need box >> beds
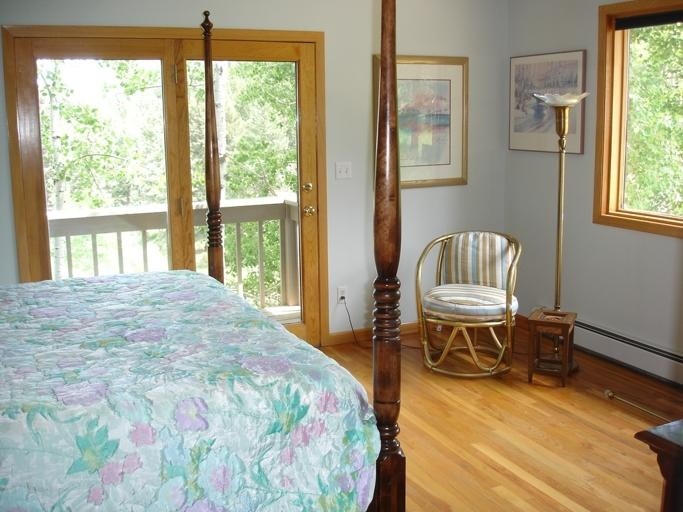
[0,2,407,512]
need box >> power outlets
[335,284,347,305]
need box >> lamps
[527,87,589,381]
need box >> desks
[633,418,682,512]
[526,307,577,387]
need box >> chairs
[414,229,523,381]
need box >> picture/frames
[370,53,471,192]
[507,49,586,155]
